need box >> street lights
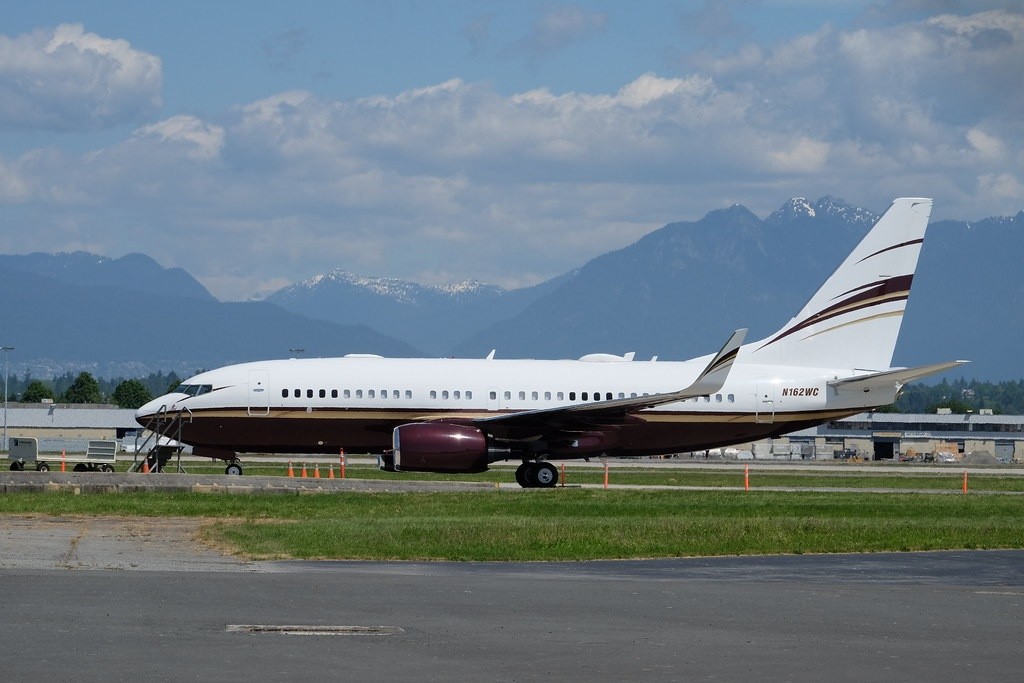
[0,345,16,452]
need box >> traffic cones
[143,455,149,473]
[327,462,335,478]
[288,459,295,477]
[313,461,320,477]
[301,461,309,478]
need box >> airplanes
[132,196,972,490]
